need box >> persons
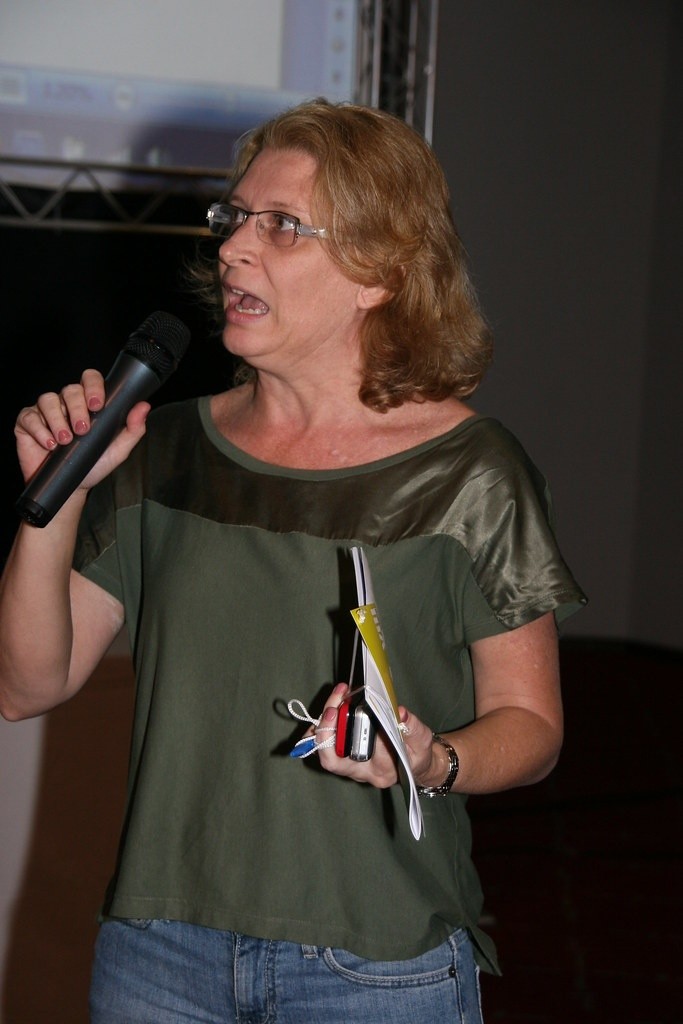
[0,99,589,1024]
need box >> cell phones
[337,696,357,757]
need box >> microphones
[16,311,190,528]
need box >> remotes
[352,698,378,762]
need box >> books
[353,547,426,842]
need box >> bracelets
[418,734,460,798]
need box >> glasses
[206,203,331,248]
